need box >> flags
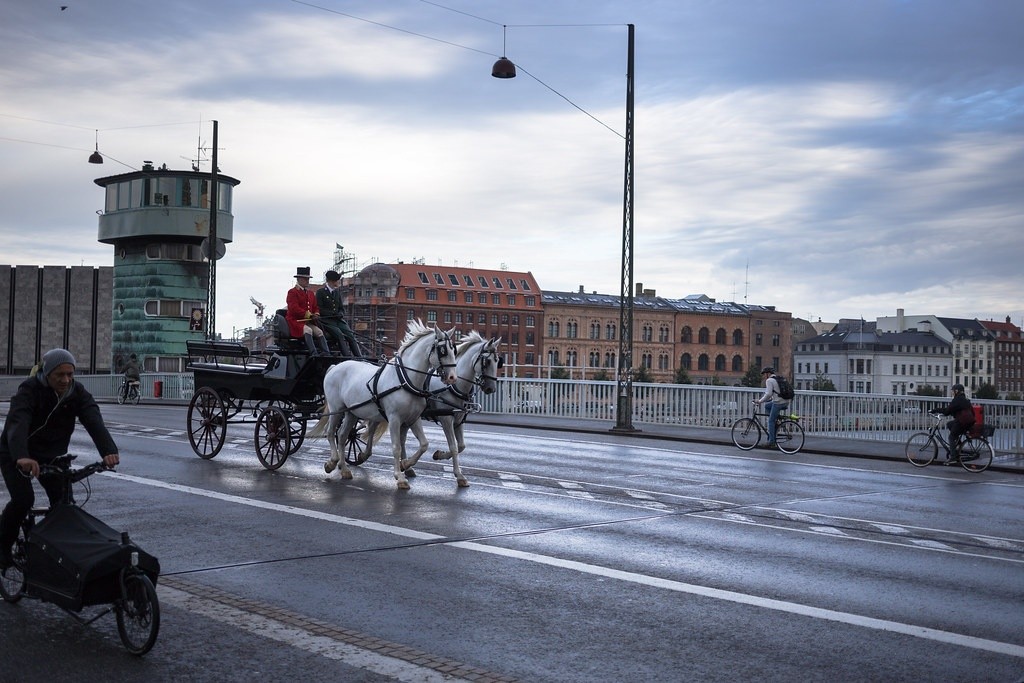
[337,243,343,249]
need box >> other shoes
[0,546,14,569]
[763,444,776,449]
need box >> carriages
[184,318,501,495]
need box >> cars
[905,406,921,413]
[712,401,737,412]
[515,401,541,413]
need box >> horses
[359,329,502,488]
[307,317,458,488]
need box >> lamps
[88,152,103,164]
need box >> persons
[120,353,141,398]
[0,347,120,571]
[754,367,789,447]
[316,271,362,357]
[286,267,330,355]
[927,384,976,464]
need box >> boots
[336,333,362,357]
[303,333,331,357]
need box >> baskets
[973,424,995,437]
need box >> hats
[760,367,773,374]
[326,271,341,282]
[294,267,313,278]
[43,349,75,377]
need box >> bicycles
[905,410,996,474]
[731,402,805,456]
[115,372,141,406]
[0,460,160,658]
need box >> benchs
[185,309,336,374]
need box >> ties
[332,291,334,298]
[304,288,307,292]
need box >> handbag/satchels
[272,314,289,336]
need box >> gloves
[338,312,344,318]
[310,314,318,326]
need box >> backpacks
[764,376,794,399]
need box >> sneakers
[944,459,961,466]
[123,394,127,398]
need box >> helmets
[950,384,964,392]
[129,353,137,359]
